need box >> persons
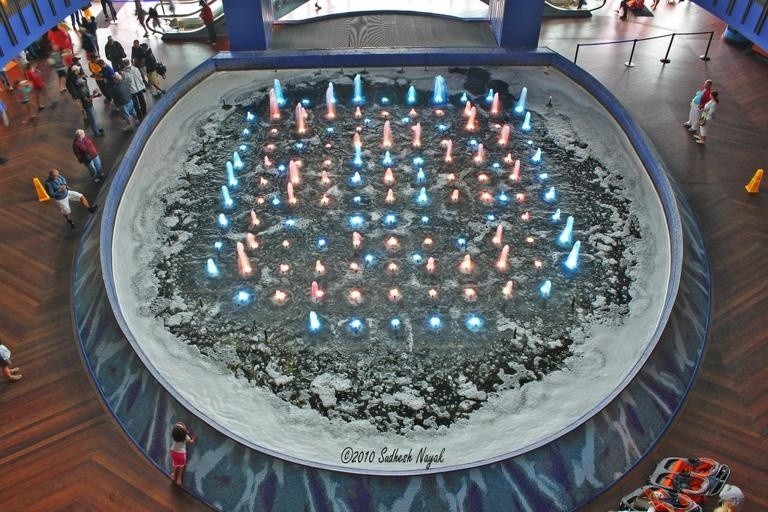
[0,341,22,380]
[72,129,105,184]
[198,0,216,46]
[168,421,196,486]
[693,90,719,144]
[619,0,628,22]
[44,167,98,229]
[0,1,168,139]
[713,483,745,512]
[682,79,713,131]
[577,0,587,10]
[650,0,660,11]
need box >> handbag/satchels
[155,62,167,74]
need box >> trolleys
[619,454,731,511]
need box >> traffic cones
[32,177,50,203]
[744,164,763,194]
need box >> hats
[71,27,148,85]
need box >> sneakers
[135,120,140,126]
[152,90,161,96]
[90,204,98,214]
[161,89,166,94]
[100,173,104,176]
[22,114,36,124]
[94,178,100,182]
[60,87,67,92]
[38,105,45,110]
[68,219,74,229]
[123,125,133,132]
[51,100,59,105]
[95,134,104,137]
[685,123,708,150]
[99,127,104,133]
